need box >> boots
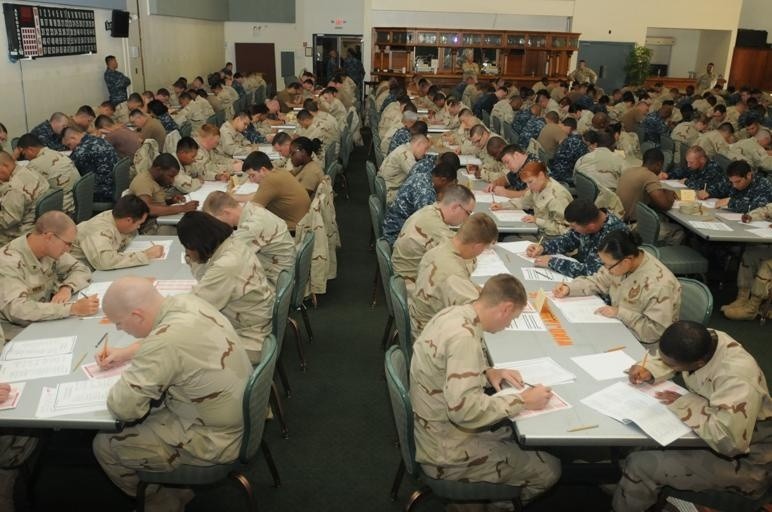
[445,499,484,512]
[142,483,195,511]
[719,286,761,322]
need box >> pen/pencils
[746,205,751,223]
[638,348,650,385]
[530,236,543,258]
[102,336,107,361]
[703,182,707,200]
[95,332,108,349]
[603,345,627,353]
[73,352,88,372]
[188,193,193,202]
[80,292,88,299]
[521,380,535,388]
[535,271,553,280]
[492,191,495,204]
[568,423,599,432]
[150,240,155,246]
[505,254,511,263]
[558,279,564,297]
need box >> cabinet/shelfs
[365,26,583,81]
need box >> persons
[0,52,367,512]
[375,59,772,512]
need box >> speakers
[111,8,129,38]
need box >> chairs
[251,268,294,409]
[0,67,772,332]
[381,343,523,511]
[128,336,281,509]
[289,232,317,365]
[663,483,745,511]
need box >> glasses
[44,230,73,249]
[602,253,631,272]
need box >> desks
[1,93,317,433]
[390,98,717,451]
